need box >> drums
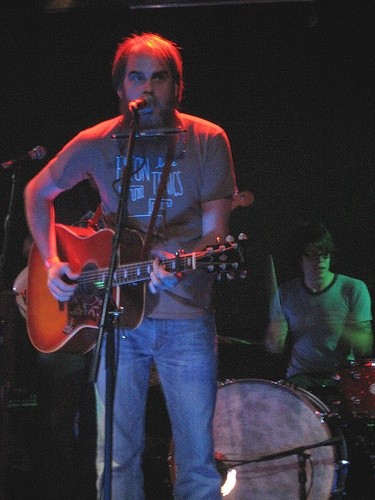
[167,376,351,500]
[330,358,375,424]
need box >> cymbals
[216,335,266,347]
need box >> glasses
[302,251,332,262]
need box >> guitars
[25,222,253,356]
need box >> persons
[25,35,240,500]
[265,223,374,380]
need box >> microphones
[0,146,47,170]
[129,94,155,112]
